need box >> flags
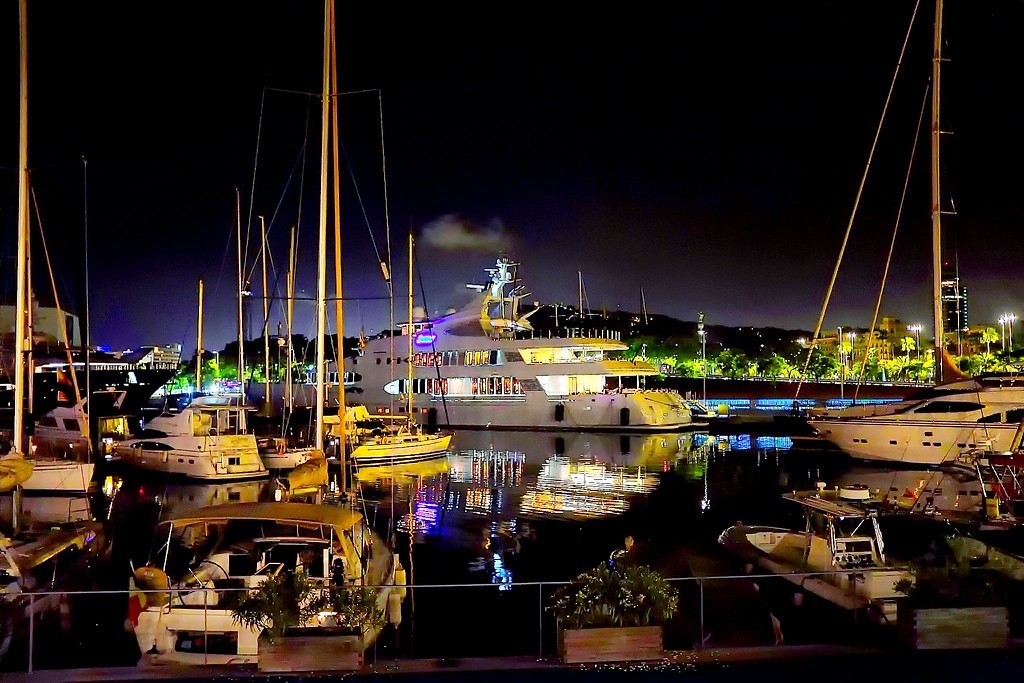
[57,369,73,402]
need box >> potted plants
[228,565,386,673]
[543,561,679,664]
[894,557,1012,650]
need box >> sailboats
[771,1,1023,469]
[0,0,454,683]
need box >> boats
[245,252,711,435]
[716,480,932,630]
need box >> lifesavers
[384,438,387,443]
[276,443,286,455]
